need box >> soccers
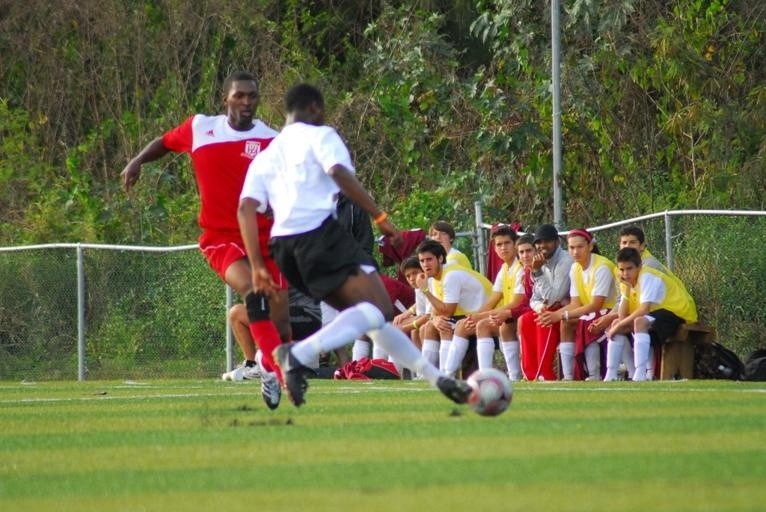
[464,368,514,417]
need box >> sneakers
[221,367,243,382]
[271,341,312,408]
[254,348,287,411]
[435,375,475,403]
[243,360,259,380]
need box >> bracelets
[408,306,416,315]
[560,307,570,321]
[373,212,387,226]
[427,317,433,320]
[421,288,429,294]
[413,321,418,330]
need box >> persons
[221,281,322,381]
[119,72,294,393]
[417,221,697,383]
[332,254,416,380]
[237,83,474,407]
[318,193,378,378]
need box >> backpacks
[742,347,765,382]
[692,339,744,380]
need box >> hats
[531,223,558,245]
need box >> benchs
[459,322,715,382]
